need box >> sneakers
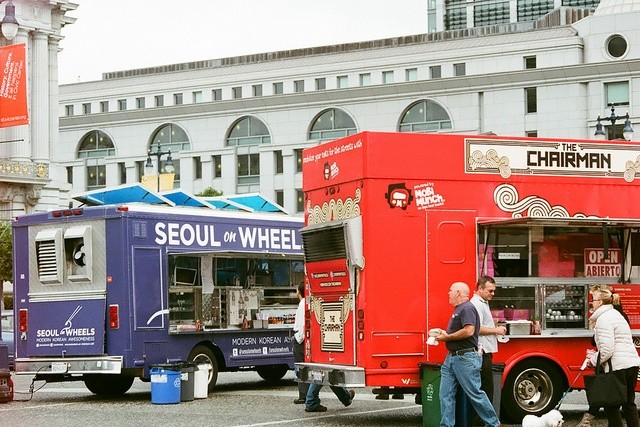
[376,396,388,399]
[294,399,306,404]
[392,395,404,399]
[305,406,327,412]
[346,390,355,406]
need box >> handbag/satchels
[583,350,627,408]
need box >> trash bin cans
[491,363,505,421]
[148,363,182,404]
[180,363,199,402]
[194,363,213,398]
[418,362,442,427]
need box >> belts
[450,347,476,356]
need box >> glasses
[487,290,497,293]
[592,297,596,302]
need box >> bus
[294,130,640,427]
[11,202,306,396]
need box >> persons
[304,383,355,411]
[470,276,507,427]
[433,282,503,427]
[586,289,639,426]
[574,283,631,427]
[293,281,308,404]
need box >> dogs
[522,409,565,427]
